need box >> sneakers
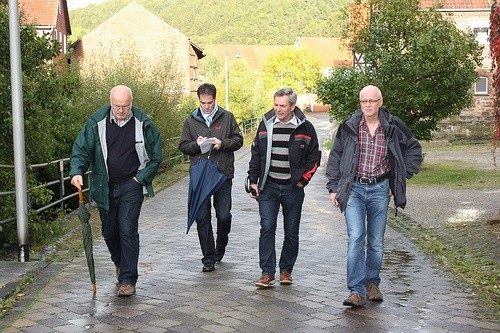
[117,283,135,296]
[343,293,366,306]
[115,268,120,279]
[255,276,276,287]
[365,283,384,301]
[279,272,292,283]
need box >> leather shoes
[215,244,226,262]
[203,262,214,272]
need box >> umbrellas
[76,181,97,294]
[244,177,258,204]
[186,143,227,236]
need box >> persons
[180,83,244,272]
[247,89,321,286]
[70,85,163,295]
[325,86,422,304]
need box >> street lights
[224,42,242,111]
[278,63,291,89]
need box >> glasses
[359,98,382,106]
[111,103,130,110]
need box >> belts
[354,171,392,185]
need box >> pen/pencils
[197,134,200,136]
